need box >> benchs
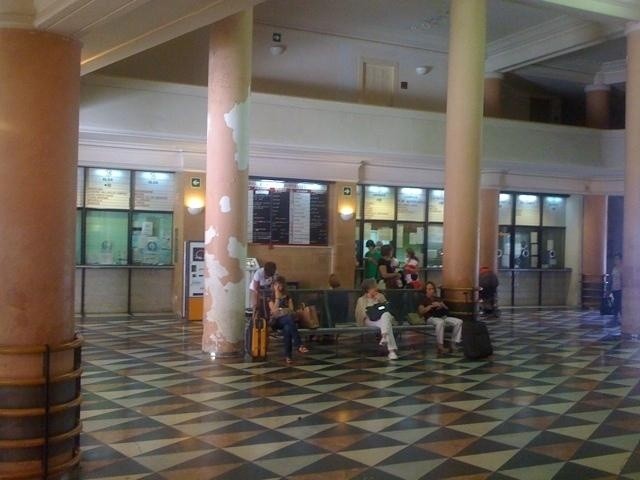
[251,286,453,357]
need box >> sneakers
[286,357,292,364]
[388,352,399,360]
[299,345,309,352]
[437,343,459,354]
[379,338,388,347]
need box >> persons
[418,281,463,354]
[250,260,281,319]
[267,276,310,363]
[356,239,499,321]
[606,253,622,317]
[354,277,399,360]
[310,274,349,344]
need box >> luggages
[246,311,267,362]
[462,293,493,359]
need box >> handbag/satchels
[600,292,618,315]
[296,302,319,328]
[407,310,425,325]
[432,307,448,318]
[404,258,420,274]
